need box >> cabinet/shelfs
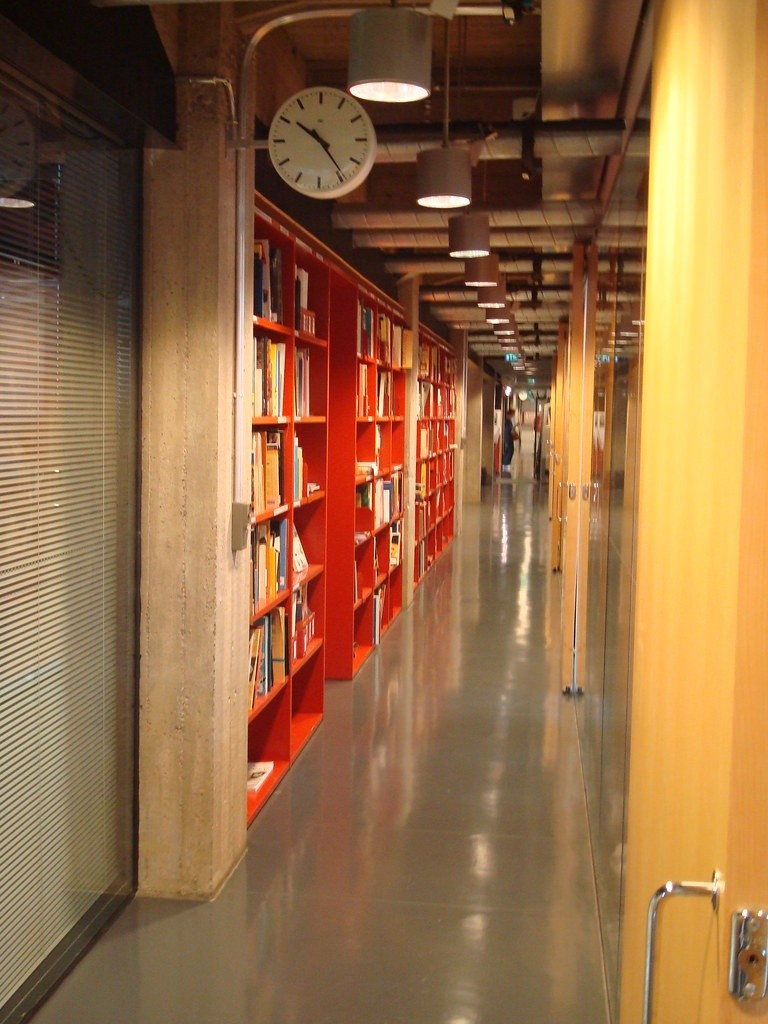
[409,318,459,589]
[244,191,332,827]
[322,260,405,679]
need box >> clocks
[267,87,378,200]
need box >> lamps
[476,274,505,308]
[485,300,509,324]
[497,325,525,370]
[347,7,432,104]
[493,313,515,336]
[464,253,498,286]
[415,16,472,209]
[448,206,489,258]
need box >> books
[250,228,455,708]
[247,761,274,791]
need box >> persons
[502,410,520,477]
